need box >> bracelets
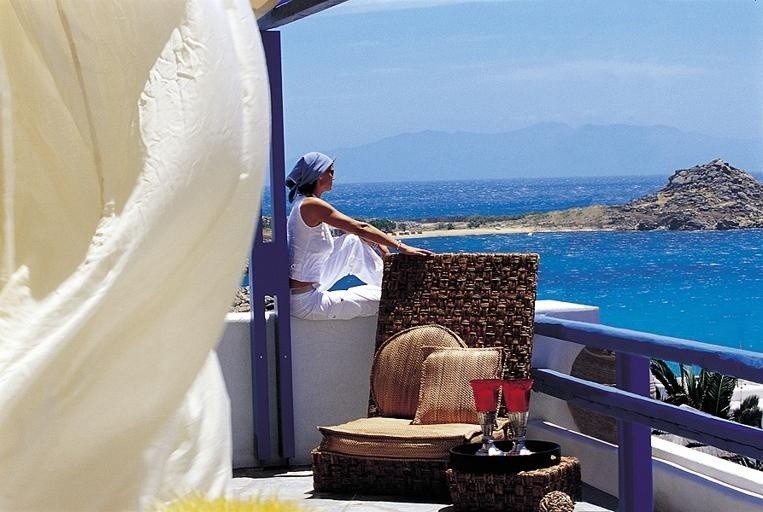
[370,243,379,250]
[396,239,402,251]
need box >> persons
[286,152,432,320]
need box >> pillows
[368,323,507,426]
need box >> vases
[567,344,656,444]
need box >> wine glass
[500,378,536,456]
[468,379,506,457]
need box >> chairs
[310,254,540,502]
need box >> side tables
[445,456,581,512]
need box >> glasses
[327,169,334,175]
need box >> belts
[291,284,319,295]
